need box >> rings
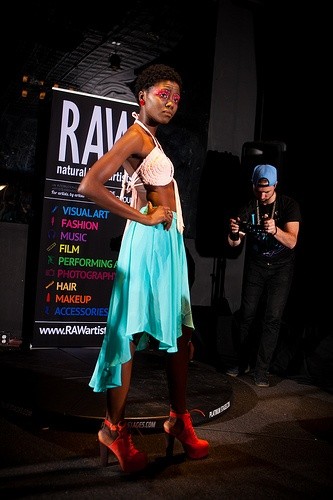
[166,210,170,214]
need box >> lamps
[22,76,76,99]
[108,42,122,71]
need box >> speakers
[195,150,245,259]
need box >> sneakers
[254,369,269,387]
[226,366,240,377]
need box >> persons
[228,164,300,387]
[79,65,208,472]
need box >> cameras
[239,221,269,240]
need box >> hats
[251,164,277,186]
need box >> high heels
[163,409,209,459]
[98,418,146,472]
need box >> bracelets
[272,226,278,237]
